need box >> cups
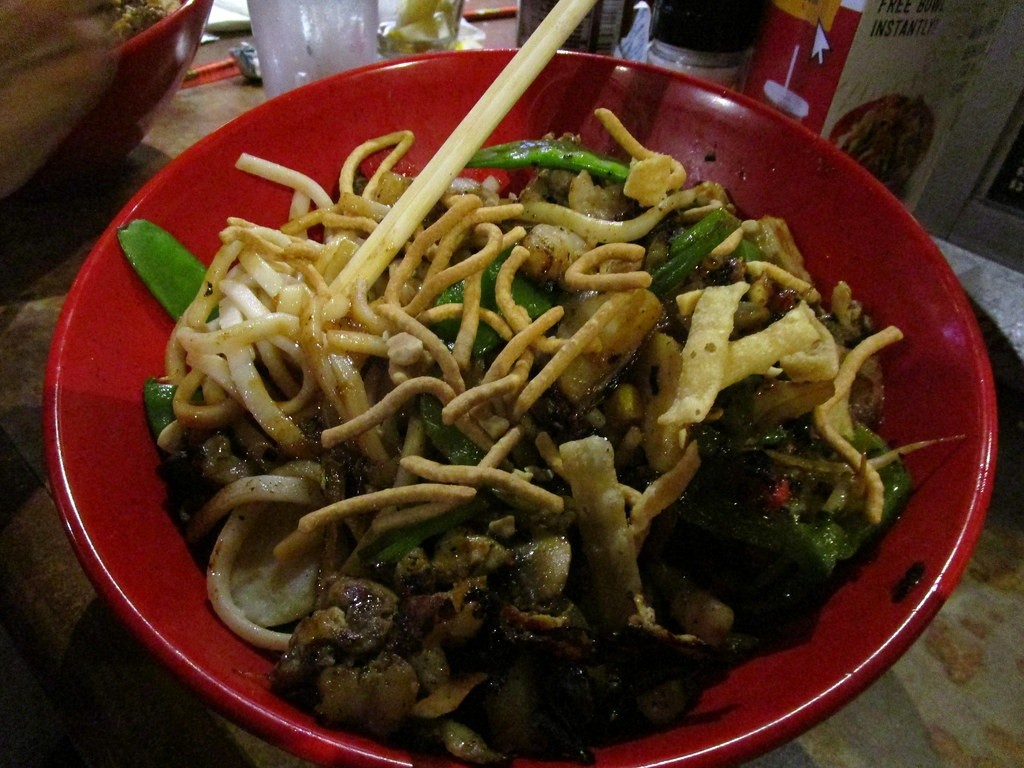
[247,0,377,101]
[379,0,465,56]
[518,0,625,56]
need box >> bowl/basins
[56,0,217,161]
[40,46,996,768]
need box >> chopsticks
[333,0,597,300]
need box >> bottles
[647,0,777,90]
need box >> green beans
[116,218,207,323]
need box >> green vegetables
[143,141,910,571]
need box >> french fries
[559,435,643,625]
